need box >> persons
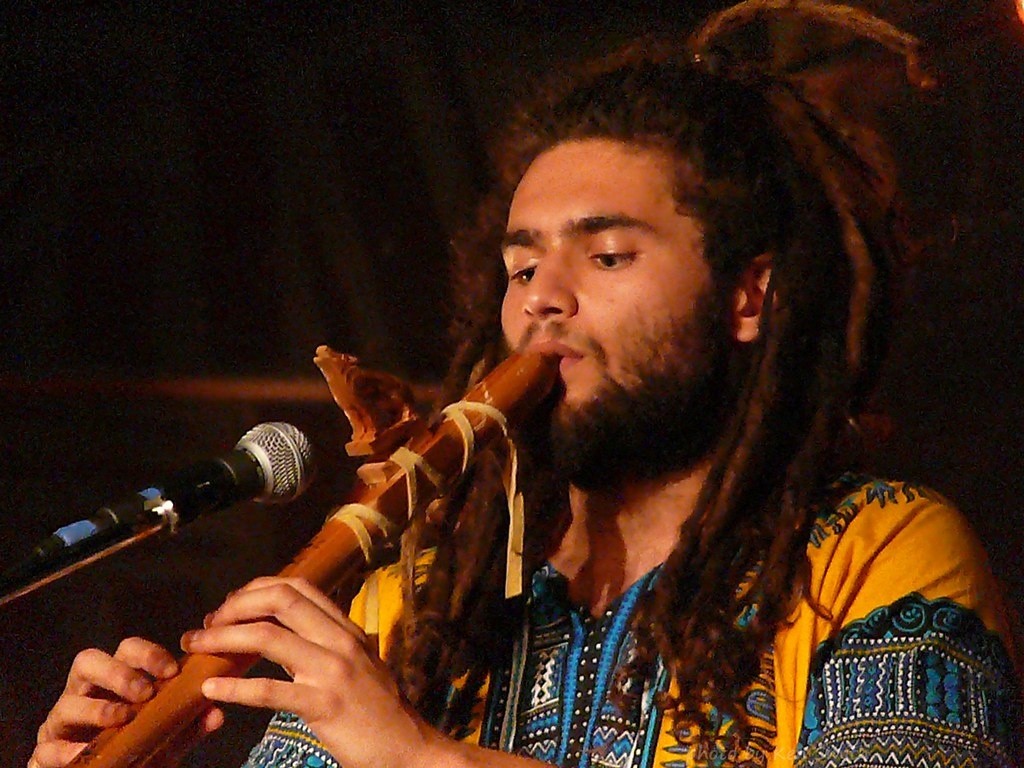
[29,0,1021,768]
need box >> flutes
[63,344,556,767]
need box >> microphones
[20,421,318,567]
